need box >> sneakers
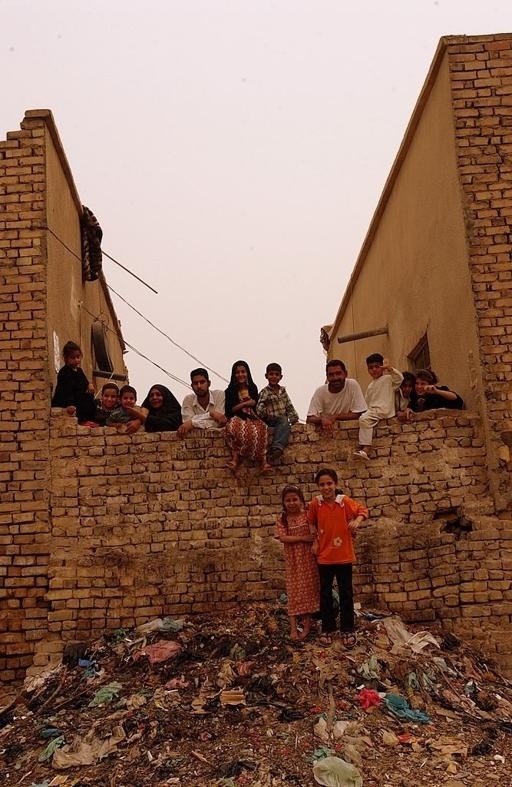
[268,448,283,461]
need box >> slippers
[259,463,274,474]
[342,631,357,648]
[317,629,334,649]
[226,459,243,468]
[352,450,371,461]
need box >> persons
[307,467,368,648]
[306,359,368,435]
[394,370,425,425]
[176,368,228,440]
[276,483,320,641]
[256,362,299,457]
[51,340,96,422]
[94,383,183,434]
[405,368,464,421]
[224,359,272,475]
[352,352,405,461]
